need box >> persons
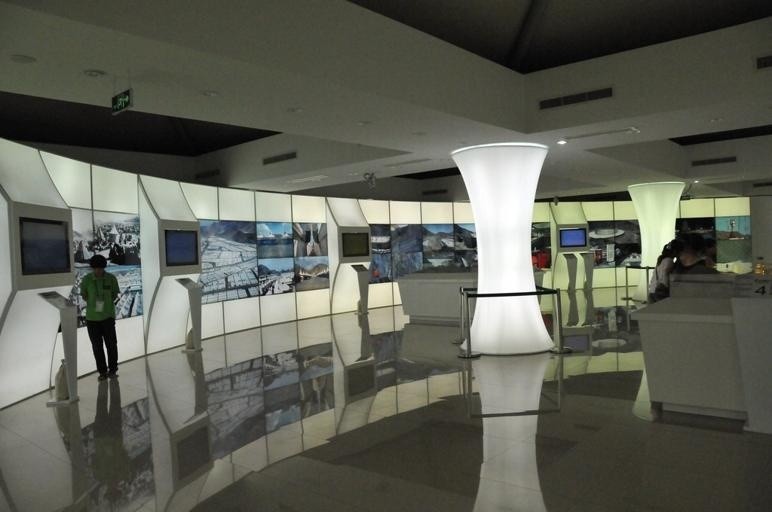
[92,380,131,501]
[647,232,716,301]
[80,254,120,380]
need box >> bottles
[754,255,766,275]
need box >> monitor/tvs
[335,225,373,264]
[156,219,202,279]
[7,199,77,292]
[556,225,588,249]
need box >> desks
[631,296,748,421]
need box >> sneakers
[98,370,118,381]
